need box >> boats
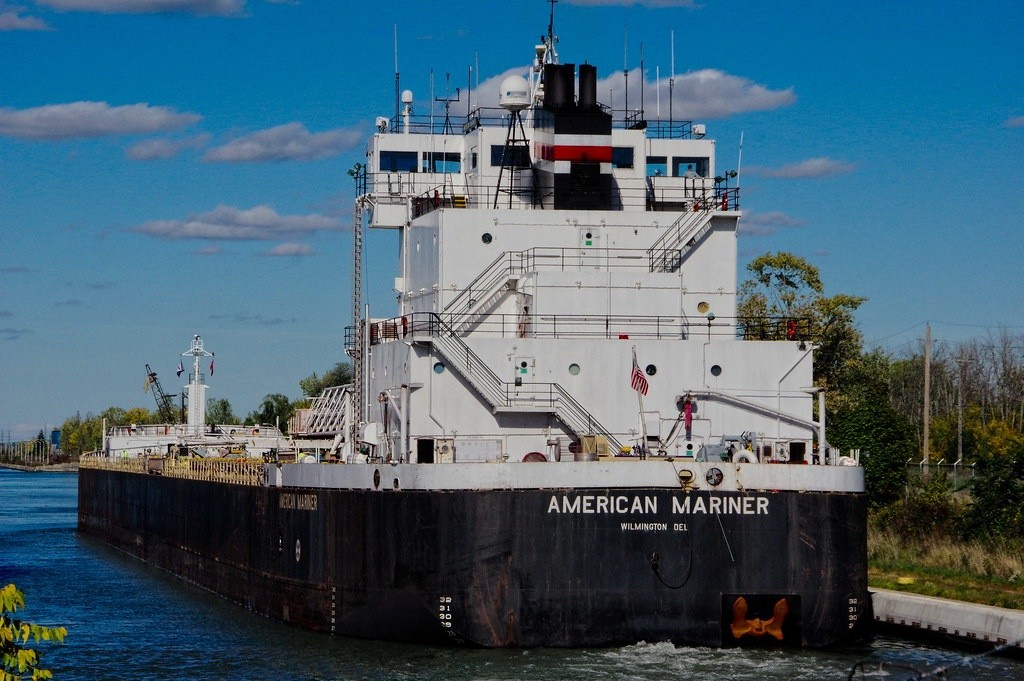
[77,5,876,655]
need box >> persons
[728,444,739,462]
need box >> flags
[631,358,649,396]
[177,361,185,378]
[210,357,214,376]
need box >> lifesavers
[732,449,758,464]
